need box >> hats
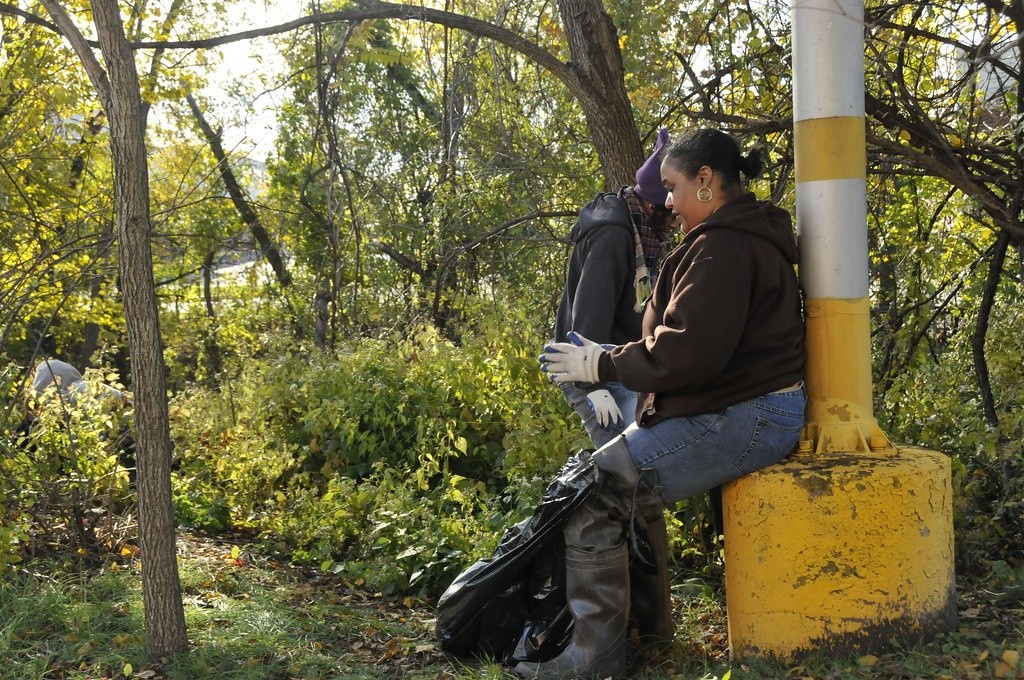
[634,128,673,205]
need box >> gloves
[538,330,607,384]
[585,388,625,432]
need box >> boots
[626,517,674,658]
[514,543,630,680]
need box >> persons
[57,367,121,477]
[513,128,808,680]
[27,349,83,460]
[554,128,682,451]
